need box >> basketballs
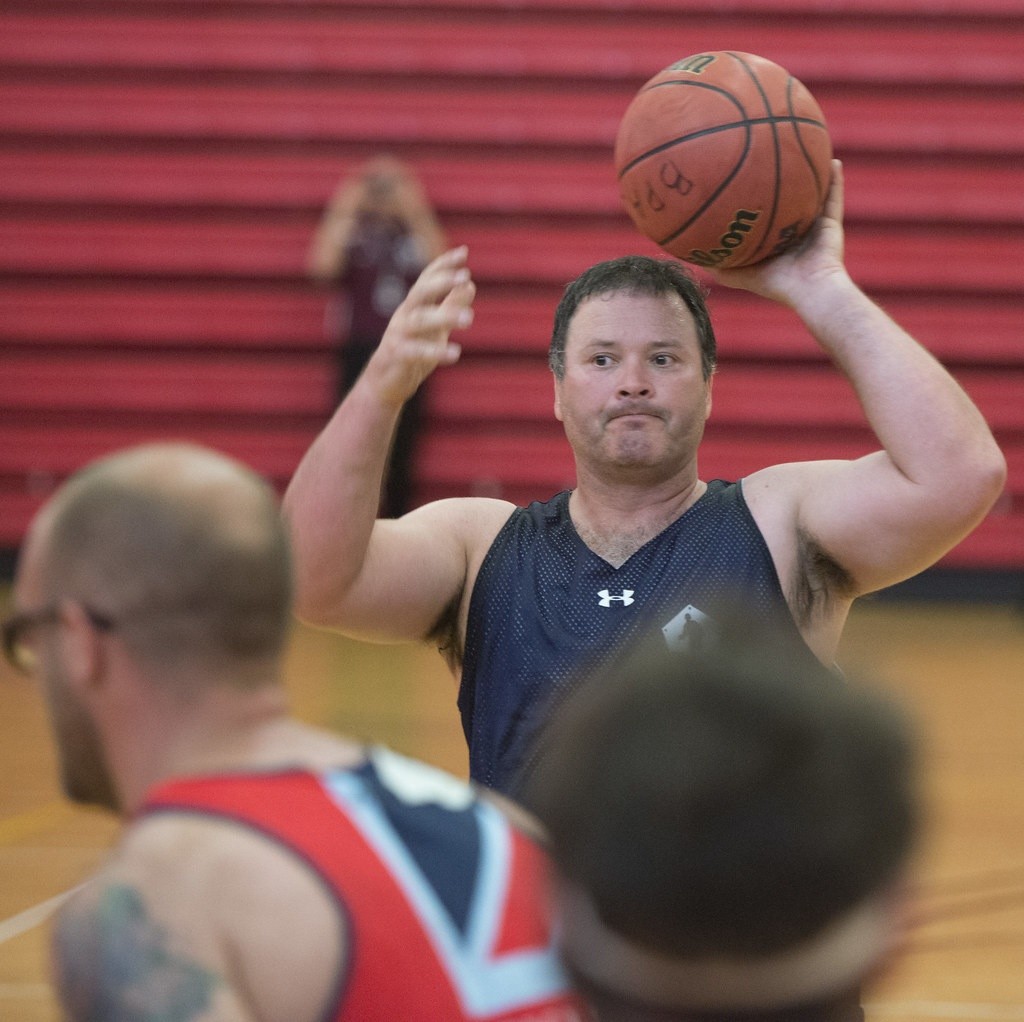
[612,48,835,268]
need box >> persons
[308,153,447,519]
[0,435,597,1022]
[531,650,924,1022]
[282,158,1008,841]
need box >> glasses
[2,606,114,675]
